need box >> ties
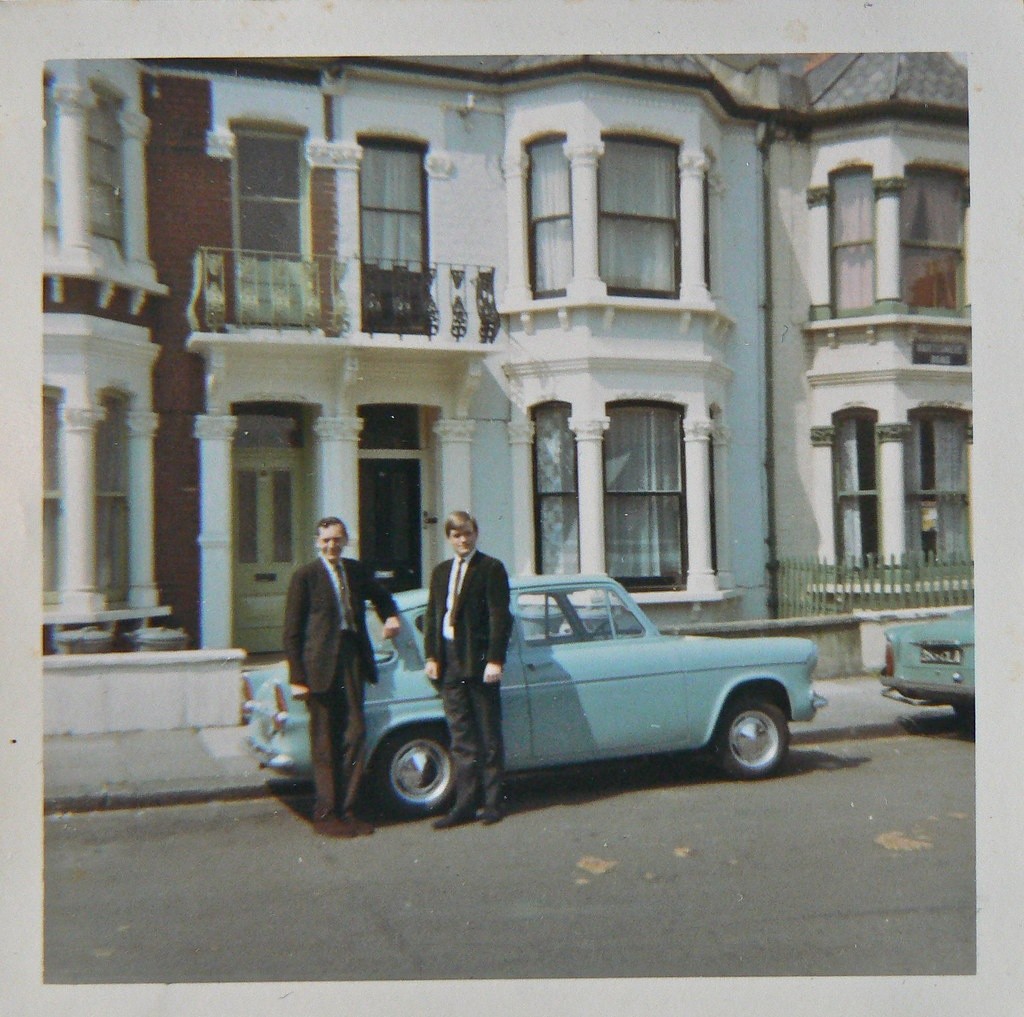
[333,564,356,634]
[450,557,465,627]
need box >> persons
[424,511,512,828]
[282,517,401,838]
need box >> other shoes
[313,807,346,837]
[433,806,475,829]
[482,806,505,824]
[340,814,374,835]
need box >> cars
[879,604,976,728]
[235,572,830,823]
